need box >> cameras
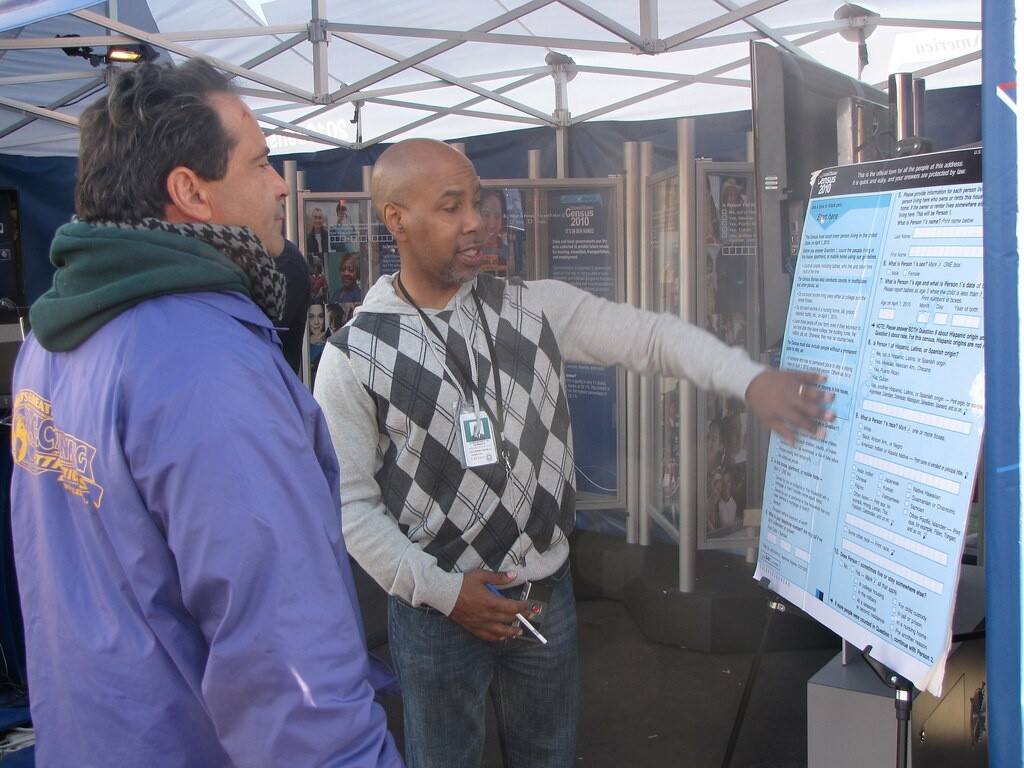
[511,581,553,642]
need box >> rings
[799,383,804,397]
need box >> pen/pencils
[486,581,549,644]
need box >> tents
[0,0,991,163]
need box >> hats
[326,302,345,315]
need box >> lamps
[54,32,100,66]
[106,41,160,64]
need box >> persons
[306,208,328,255]
[272,239,311,374]
[329,205,356,252]
[325,304,346,340]
[331,254,362,302]
[309,304,326,372]
[472,425,483,441]
[10,59,404,768]
[478,190,508,278]
[312,258,328,303]
[313,138,835,768]
